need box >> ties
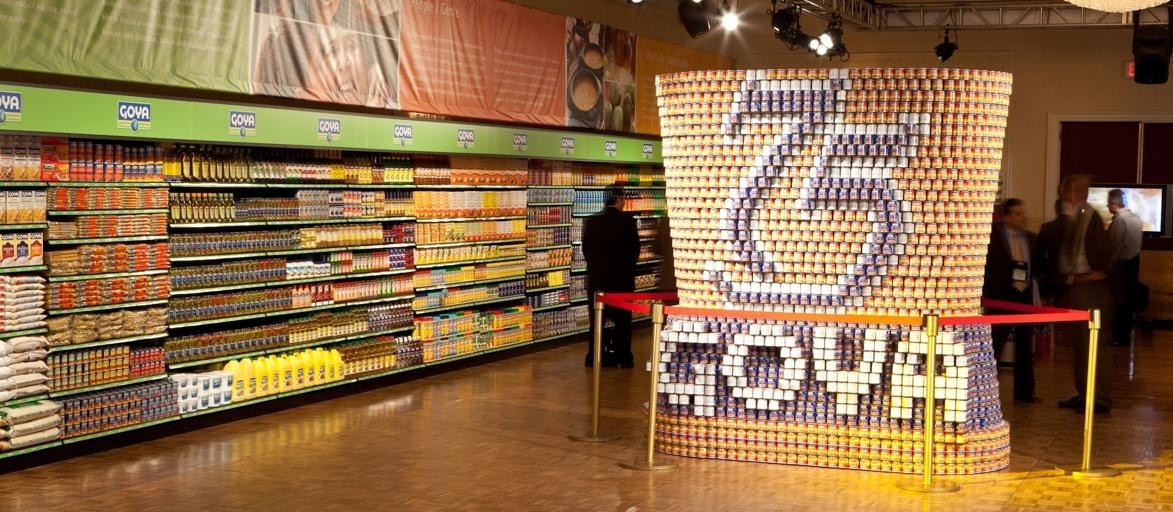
[1012,237,1029,293]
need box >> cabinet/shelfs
[0,130,679,460]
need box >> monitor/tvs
[1086,185,1167,238]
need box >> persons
[649,204,678,328]
[253,20,398,109]
[1057,174,1118,418]
[580,183,635,369]
[982,198,1047,404]
[1104,188,1144,347]
[277,0,347,28]
[1035,200,1074,308]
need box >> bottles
[162,259,287,288]
[572,166,668,263]
[524,203,576,310]
[383,191,415,217]
[167,191,297,222]
[289,222,425,381]
[164,287,288,361]
[167,231,291,259]
[171,140,451,187]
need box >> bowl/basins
[580,43,605,70]
[567,68,602,117]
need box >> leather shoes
[1058,394,1112,417]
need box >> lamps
[676,1,852,60]
[931,27,960,63]
